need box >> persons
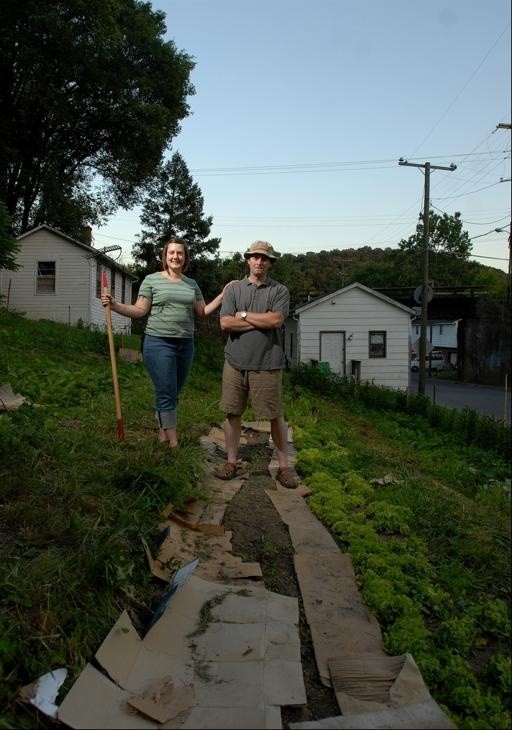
[215,241,300,490]
[101,237,239,449]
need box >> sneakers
[276,467,299,489]
[215,462,239,481]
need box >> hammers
[103,272,137,449]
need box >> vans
[411,352,445,372]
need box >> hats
[243,240,281,263]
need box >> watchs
[241,310,247,321]
